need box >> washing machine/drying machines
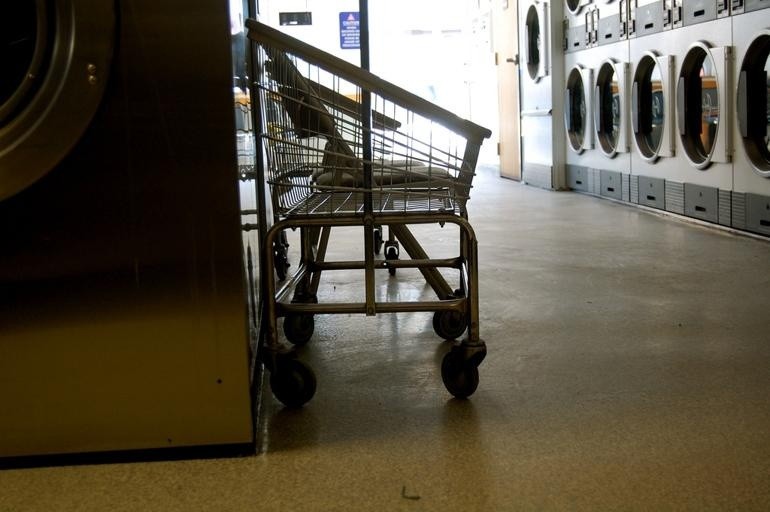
[0,0,262,459]
[495,1,770,236]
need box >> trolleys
[243,16,493,408]
